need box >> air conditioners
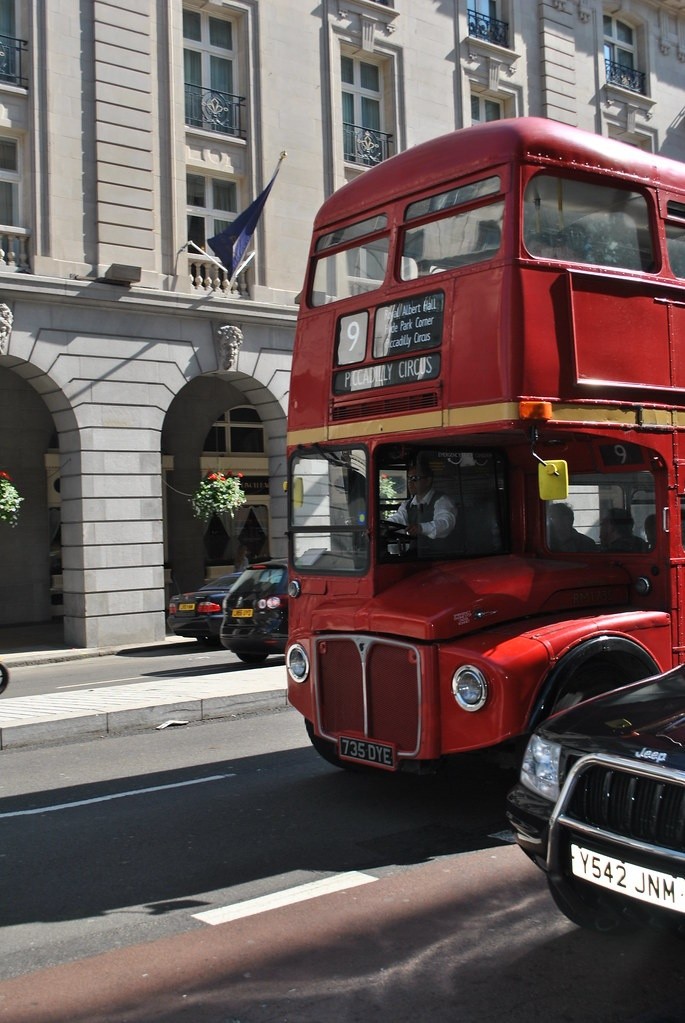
[44,452,61,509]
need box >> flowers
[0,471,25,527]
[188,467,247,523]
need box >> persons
[644,513,657,551]
[523,219,607,267]
[233,544,249,573]
[547,502,597,553]
[601,507,648,553]
[365,464,461,559]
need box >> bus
[286,117,685,827]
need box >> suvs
[502,659,685,938]
[221,553,366,662]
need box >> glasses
[408,475,428,481]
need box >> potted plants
[205,559,236,579]
[164,561,175,584]
[51,564,63,588]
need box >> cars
[167,572,247,647]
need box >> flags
[206,168,280,281]
